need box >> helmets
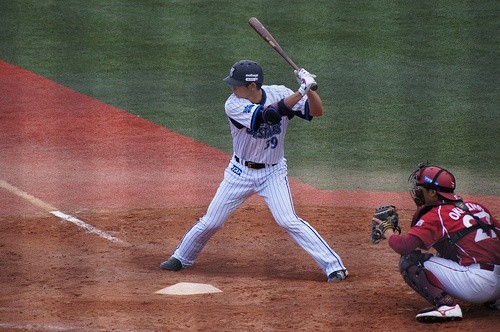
[408,165,462,207]
[223,60,263,85]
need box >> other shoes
[327,269,349,283]
[160,257,182,272]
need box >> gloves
[294,68,310,85]
[298,74,317,96]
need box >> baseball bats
[248,16,319,92]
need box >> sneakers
[415,304,462,324]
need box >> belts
[235,155,278,169]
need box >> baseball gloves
[371,205,401,244]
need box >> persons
[160,60,348,283]
[372,165,500,324]
